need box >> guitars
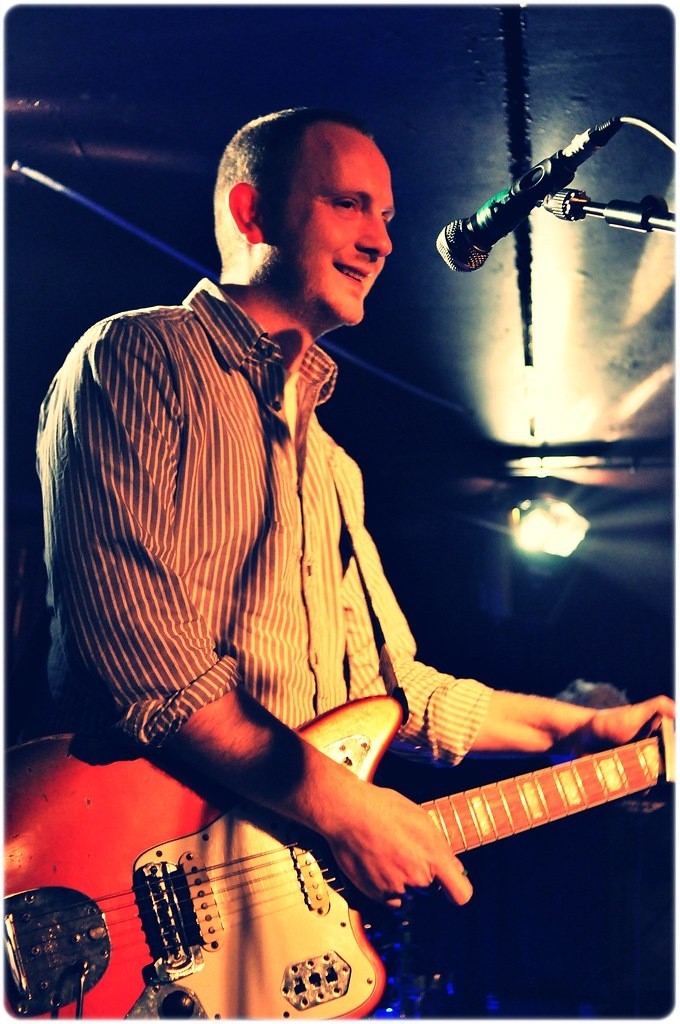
[4,692,675,1019]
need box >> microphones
[436,116,622,273]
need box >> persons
[36,108,676,908]
